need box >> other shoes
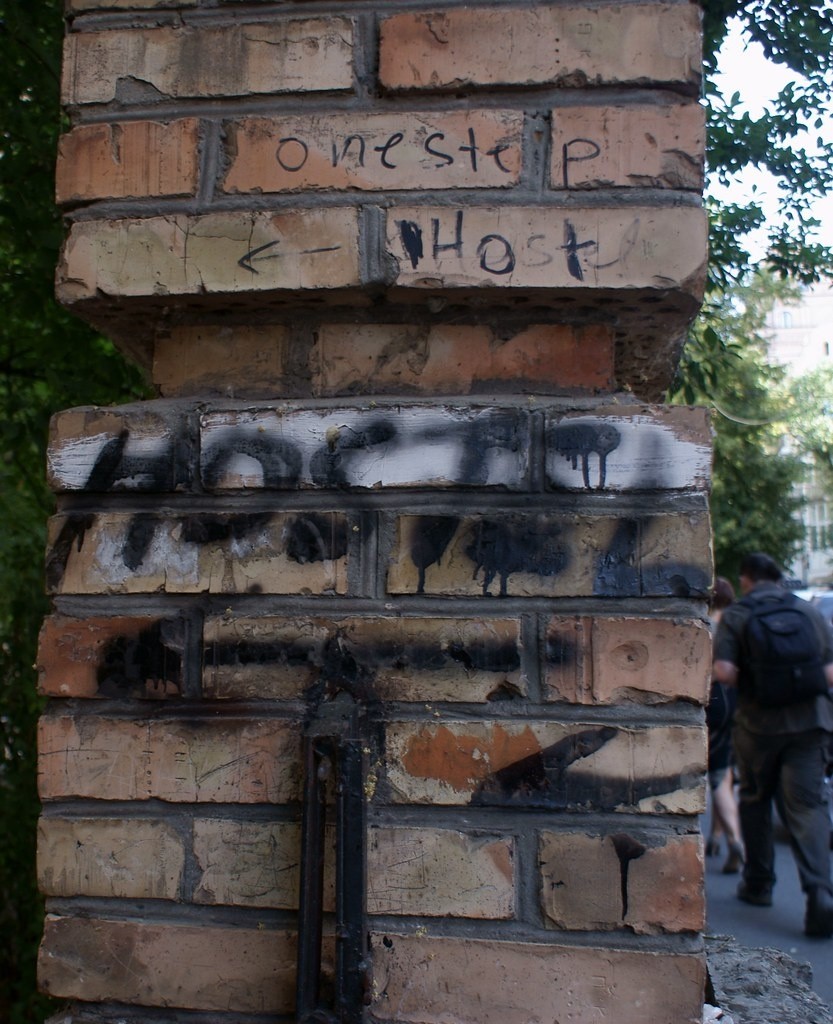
[737,881,771,905]
[806,889,833,935]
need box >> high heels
[706,836,718,858]
[723,842,746,873]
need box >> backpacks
[736,593,827,705]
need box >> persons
[705,578,746,874]
[713,553,833,940]
